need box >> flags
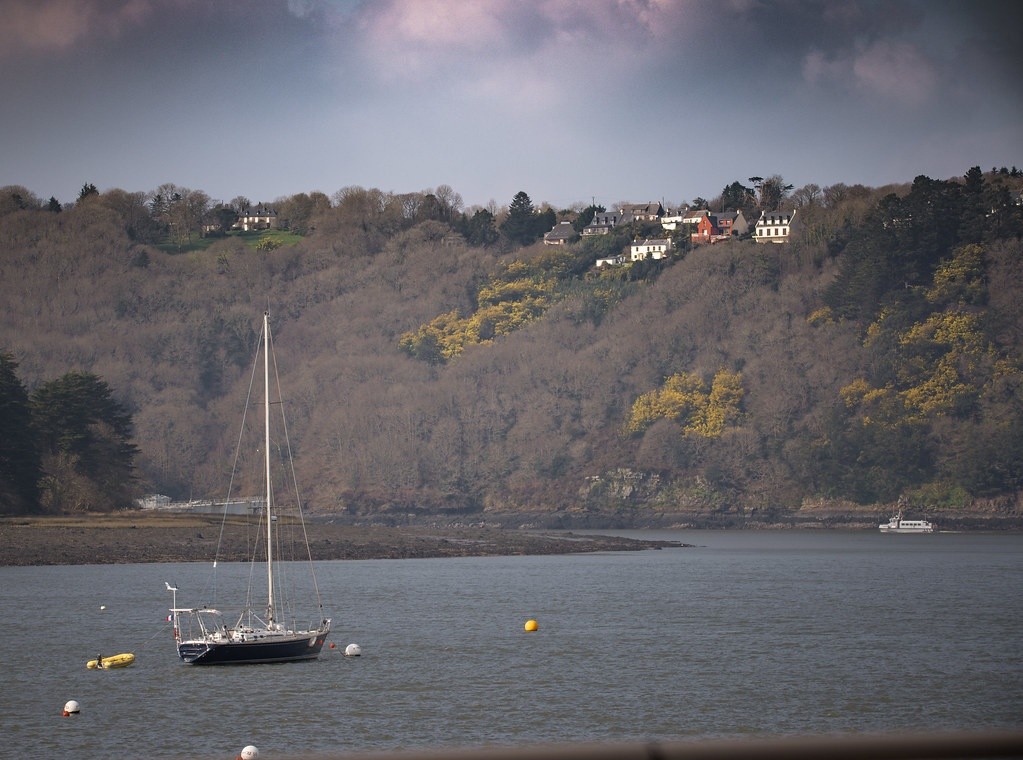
[165,614,174,621]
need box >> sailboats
[164,296,332,667]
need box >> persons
[238,626,263,644]
[97,653,104,667]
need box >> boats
[138,494,266,516]
[879,505,933,533]
[87,654,136,670]
